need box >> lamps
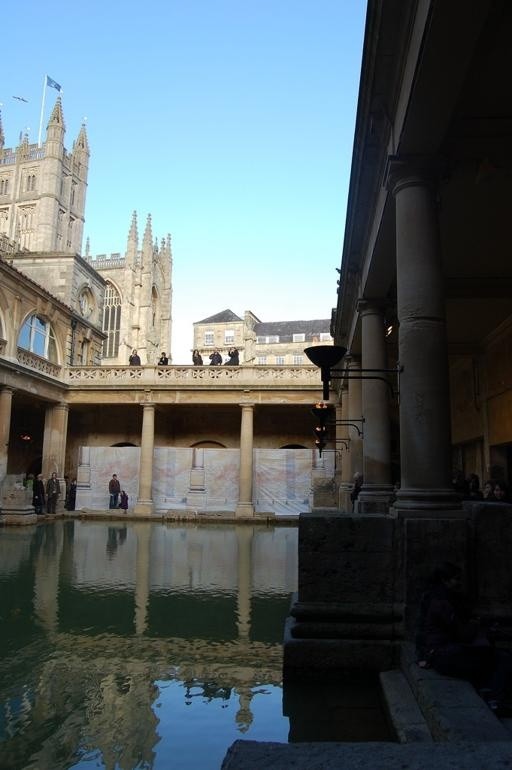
[303,346,405,458]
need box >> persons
[65,476,76,511]
[224,347,239,365]
[185,687,231,709]
[119,490,128,509]
[158,352,168,366]
[351,472,363,513]
[193,350,203,365]
[46,471,61,514]
[129,350,140,366]
[210,351,222,366]
[26,474,35,489]
[393,480,401,493]
[452,462,508,502]
[106,526,128,560]
[32,474,44,514]
[109,474,120,508]
[29,522,75,561]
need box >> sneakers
[488,700,505,714]
